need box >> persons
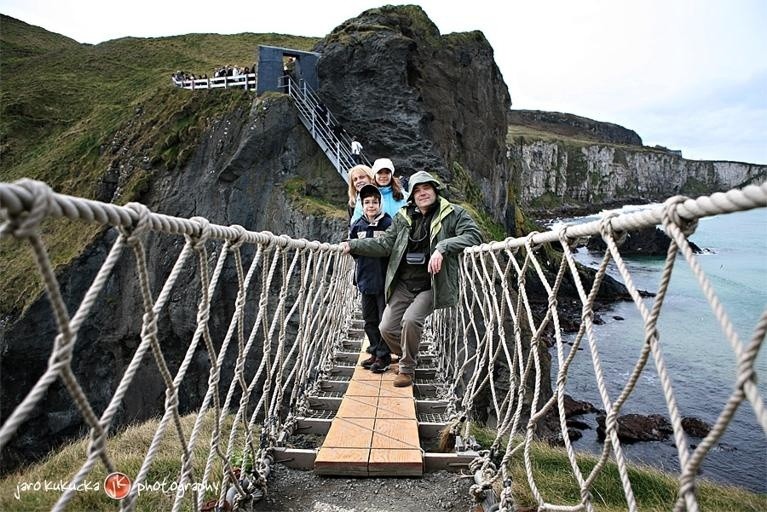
[351,136,363,164]
[172,64,256,89]
[340,158,483,387]
[286,57,295,69]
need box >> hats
[371,159,394,178]
[360,184,384,207]
[406,170,440,202]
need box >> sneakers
[371,356,391,372]
[362,355,376,368]
[393,373,413,386]
